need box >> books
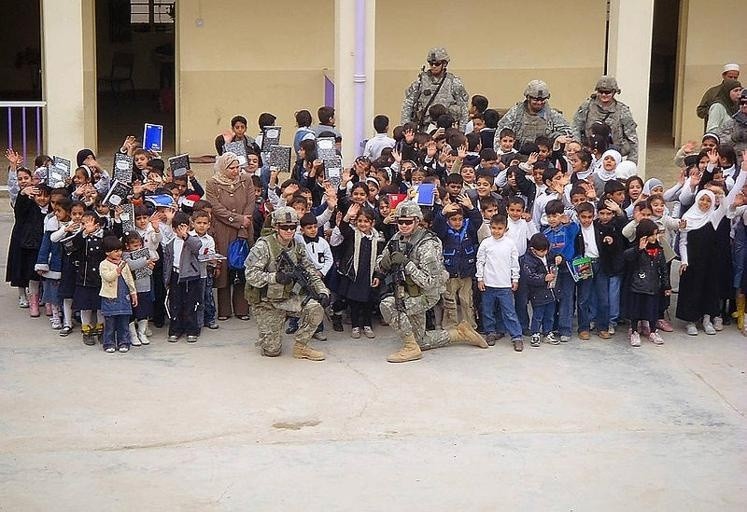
[143,122,162,153]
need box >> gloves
[318,292,330,308]
[391,252,409,266]
[275,271,292,286]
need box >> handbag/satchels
[227,238,251,271]
[381,252,393,271]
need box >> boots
[137,320,150,345]
[28,293,41,317]
[44,302,53,316]
[129,321,141,346]
[387,333,422,364]
[448,320,489,349]
[293,341,326,361]
[735,294,746,332]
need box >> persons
[242,205,326,363]
[207,97,502,338]
[705,80,744,143]
[697,64,740,135]
[5,136,221,353]
[493,79,572,153]
[572,75,638,165]
[374,201,489,363]
[715,89,747,166]
[474,124,745,353]
[401,47,470,134]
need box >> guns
[388,240,405,332]
[411,64,426,124]
[276,249,334,325]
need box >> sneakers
[284,316,376,341]
[147,313,250,343]
[486,314,731,351]
[18,290,129,353]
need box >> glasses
[279,224,298,231]
[738,100,747,106]
[396,217,416,225]
[598,89,612,94]
[428,62,443,67]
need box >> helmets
[741,89,747,99]
[271,206,298,226]
[427,47,450,65]
[524,79,551,100]
[395,199,424,223]
[595,76,621,94]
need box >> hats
[76,149,96,166]
[721,63,740,76]
